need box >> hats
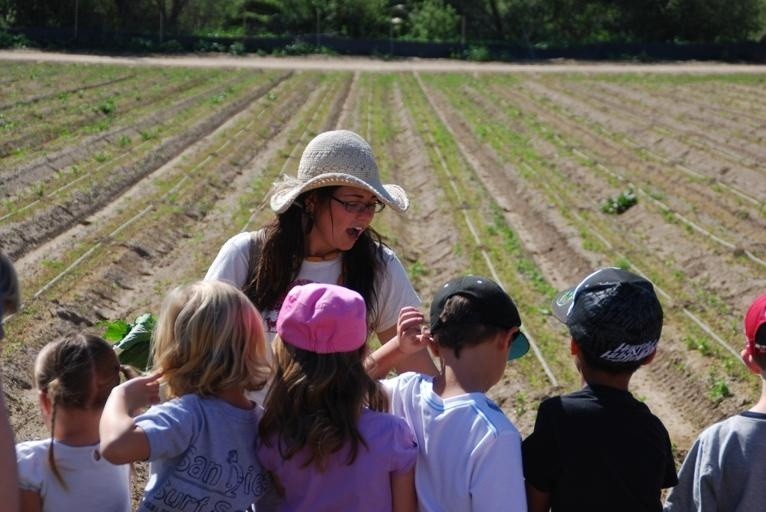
[745,295,766,353]
[430,275,530,361]
[270,129,409,214]
[277,283,367,354]
[550,268,663,368]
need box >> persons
[522,267,679,512]
[254,283,420,512]
[14,330,143,512]
[663,293,766,512]
[98,281,275,512]
[363,275,530,512]
[201,130,440,408]
[0,248,20,512]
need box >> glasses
[332,200,388,215]
